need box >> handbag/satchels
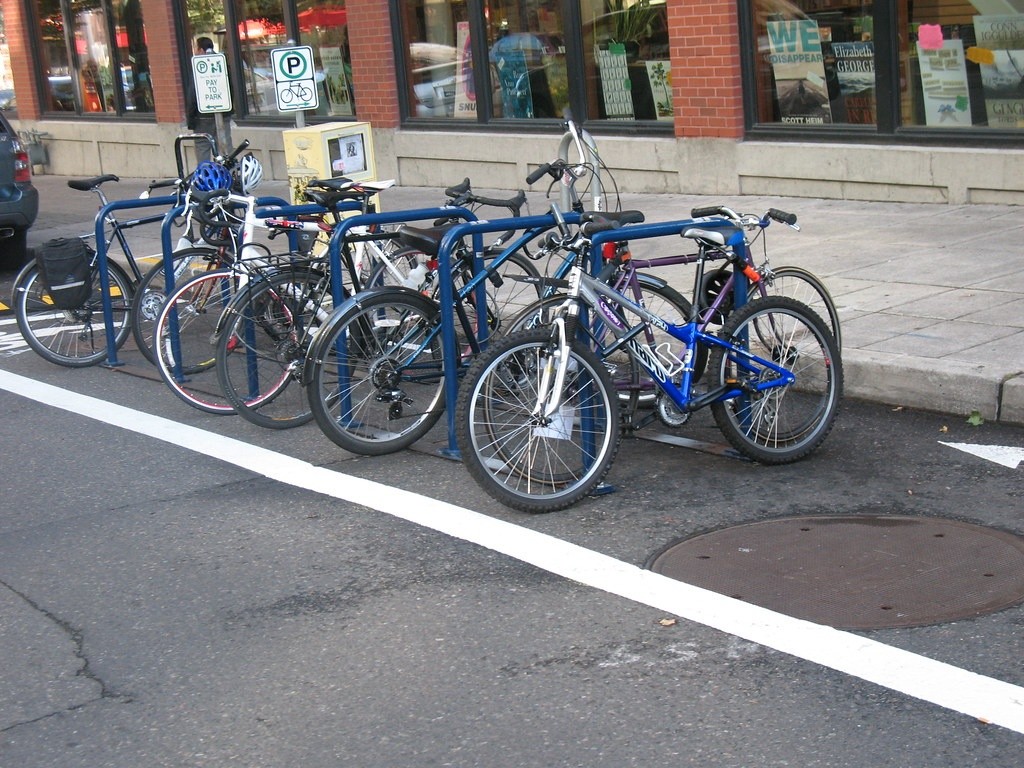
[36,237,92,310]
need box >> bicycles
[10,137,845,516]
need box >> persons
[85,59,106,112]
[341,27,356,115]
[185,37,235,165]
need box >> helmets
[242,156,263,196]
[190,160,233,191]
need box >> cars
[489,29,564,77]
[243,67,277,112]
[48,75,76,111]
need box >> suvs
[0,111,39,273]
[407,57,503,122]
